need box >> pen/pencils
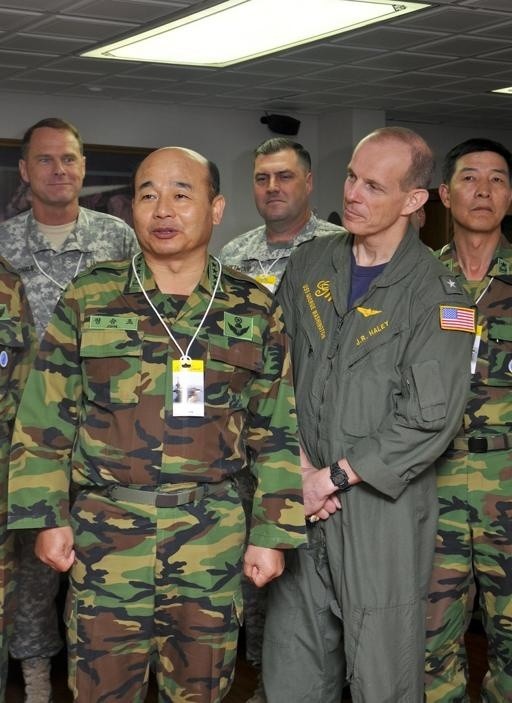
[496,338,499,343]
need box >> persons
[1,116,142,703]
[0,254,35,703]
[259,124,476,703]
[216,137,350,703]
[411,205,426,238]
[7,146,311,702]
[425,136,511,702]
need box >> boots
[22,657,52,703]
[246,681,266,703]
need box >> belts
[104,480,230,507]
[451,434,512,452]
